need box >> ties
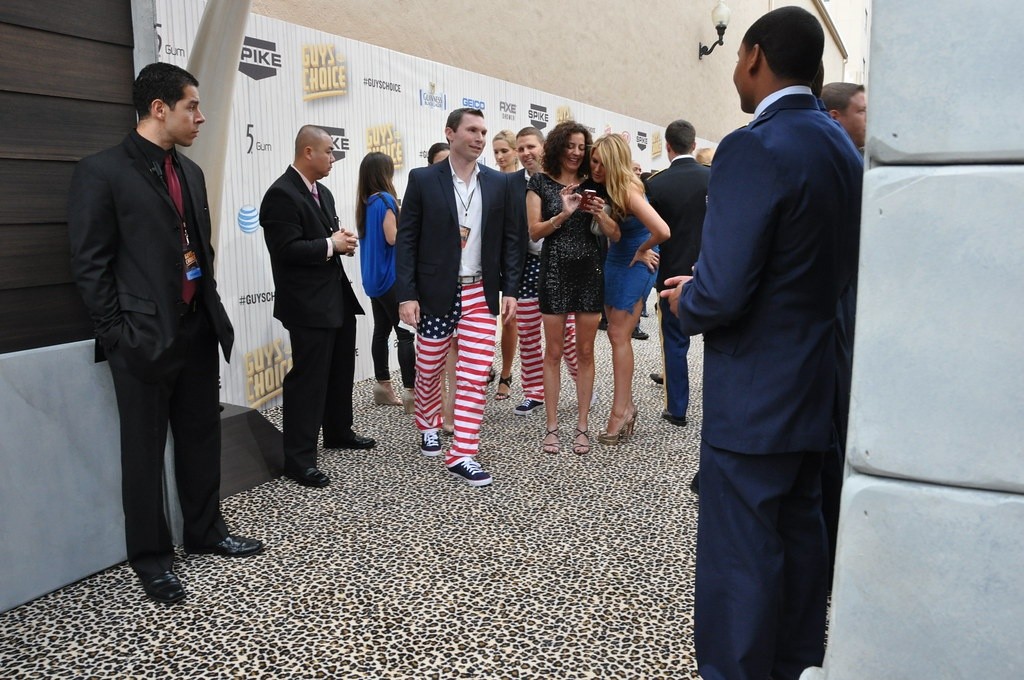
[164,153,195,304]
[311,184,320,208]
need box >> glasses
[632,167,641,172]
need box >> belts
[174,301,203,317]
[457,276,482,284]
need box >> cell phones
[580,190,596,210]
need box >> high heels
[372,379,403,405]
[484,367,495,394]
[598,404,638,446]
[403,388,415,414]
[495,372,512,400]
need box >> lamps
[698,1,730,61]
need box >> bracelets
[550,217,561,229]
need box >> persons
[396,106,512,498]
[504,126,581,416]
[256,122,375,491]
[589,134,672,442]
[645,120,726,425]
[630,4,865,680]
[487,130,520,398]
[425,142,459,436]
[70,61,265,608]
[524,118,622,456]
[356,151,419,413]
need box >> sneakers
[515,397,543,416]
[419,430,442,457]
[446,457,492,486]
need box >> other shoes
[690,469,699,495]
[632,327,648,340]
[641,309,649,317]
[650,373,663,385]
[597,318,608,329]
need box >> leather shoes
[283,466,329,487]
[442,421,455,434]
[133,567,186,603]
[185,535,263,558]
[323,435,376,448]
[662,408,687,427]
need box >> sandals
[543,425,560,454]
[573,427,590,454]
